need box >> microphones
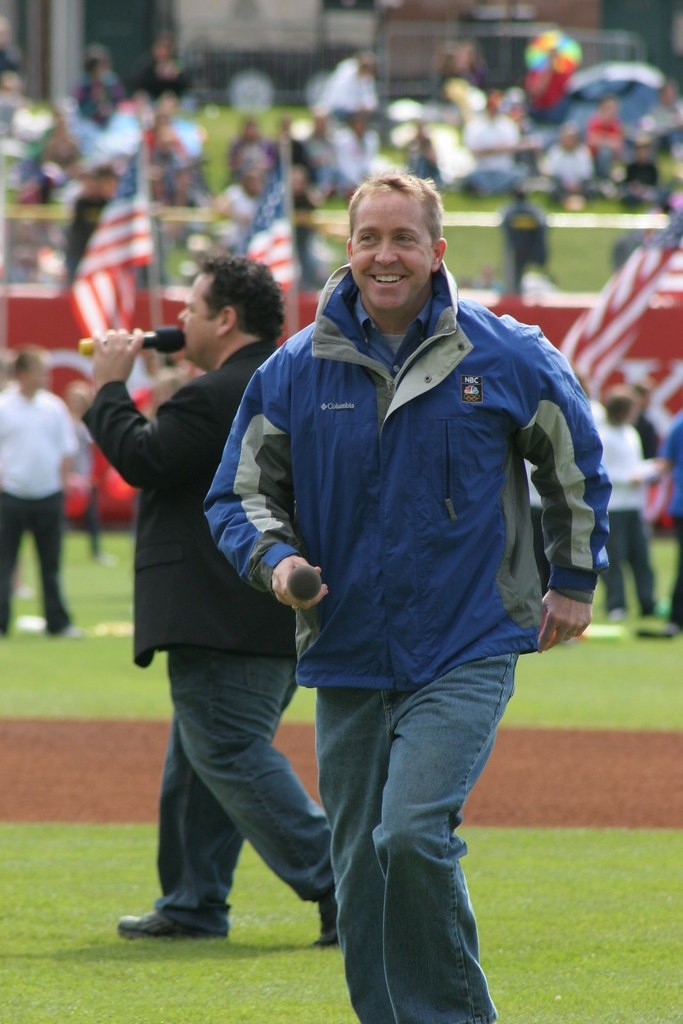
[287,565,321,600]
[80,327,187,357]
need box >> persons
[1,350,90,638]
[80,258,338,949]
[70,388,109,562]
[205,174,610,1024]
[1,21,683,294]
[523,382,683,639]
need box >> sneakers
[315,891,338,944]
[119,911,224,937]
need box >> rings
[292,606,298,609]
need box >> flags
[240,153,292,287]
[561,207,683,389]
[70,150,151,338]
